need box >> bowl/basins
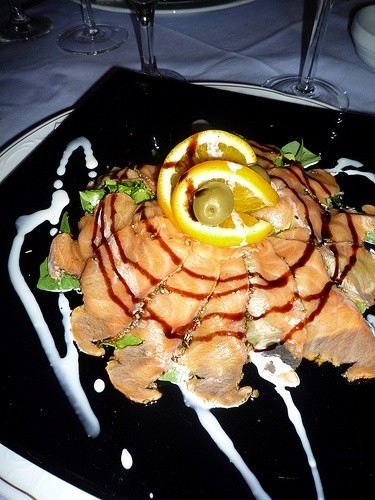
[351,5,375,69]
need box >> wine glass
[0,0,55,43]
[57,0,129,56]
[261,0,350,111]
[117,0,186,81]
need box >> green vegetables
[280,140,322,166]
[36,178,155,292]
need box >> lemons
[155,128,257,223]
[171,161,278,247]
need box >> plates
[72,1,256,15]
[0,83,373,500]
